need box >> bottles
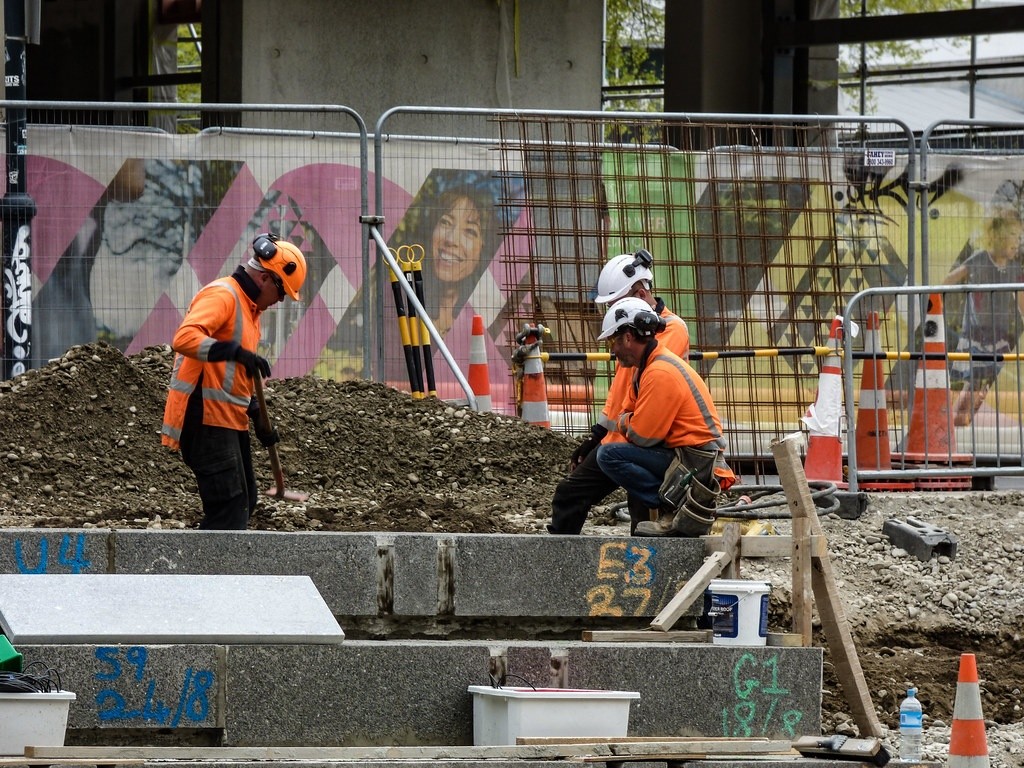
[898,689,922,762]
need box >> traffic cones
[890,294,974,491]
[804,319,853,488]
[848,310,915,492]
[467,315,495,413]
[949,653,991,768]
[520,322,553,429]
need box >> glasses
[269,271,286,302]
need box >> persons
[546,249,690,534]
[77,155,144,293]
[161,232,308,529]
[420,183,493,355]
[597,297,736,533]
[944,215,1024,424]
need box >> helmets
[595,254,655,302]
[597,297,658,341]
[258,241,307,300]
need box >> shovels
[253,370,308,501]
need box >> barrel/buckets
[707,579,770,647]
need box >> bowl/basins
[1,691,76,754]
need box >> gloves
[250,401,280,446]
[571,423,608,472]
[208,340,271,381]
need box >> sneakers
[634,512,673,536]
